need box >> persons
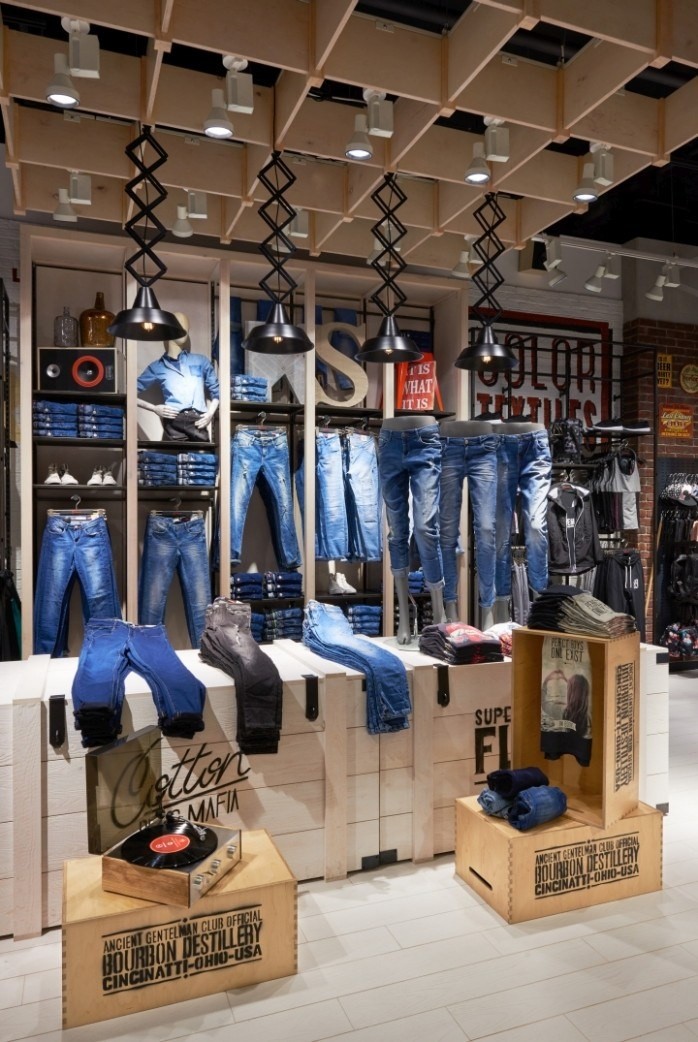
[564,672,591,735]
[381,416,552,644]
[135,310,219,442]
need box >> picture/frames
[680,364,698,394]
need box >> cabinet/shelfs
[17,223,470,660]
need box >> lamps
[239,148,315,355]
[645,262,680,302]
[345,88,393,161]
[464,115,509,185]
[171,192,208,238]
[272,209,310,253]
[44,14,102,108]
[453,191,520,373]
[452,234,485,279]
[584,253,620,293]
[543,237,568,288]
[53,174,92,222]
[107,122,188,342]
[570,142,614,203]
[203,53,254,139]
[353,171,424,363]
[366,222,401,267]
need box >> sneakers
[103,464,117,485]
[86,465,102,486]
[61,463,79,485]
[44,464,61,485]
[335,572,357,594]
[328,572,345,594]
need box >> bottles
[53,305,79,347]
[78,292,116,348]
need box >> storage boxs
[512,625,641,828]
[454,793,663,924]
[61,827,298,1030]
[101,817,242,909]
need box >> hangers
[46,494,107,526]
[317,413,379,439]
[559,468,576,484]
[661,471,698,526]
[149,497,204,523]
[601,537,640,558]
[584,438,649,465]
[235,411,286,434]
